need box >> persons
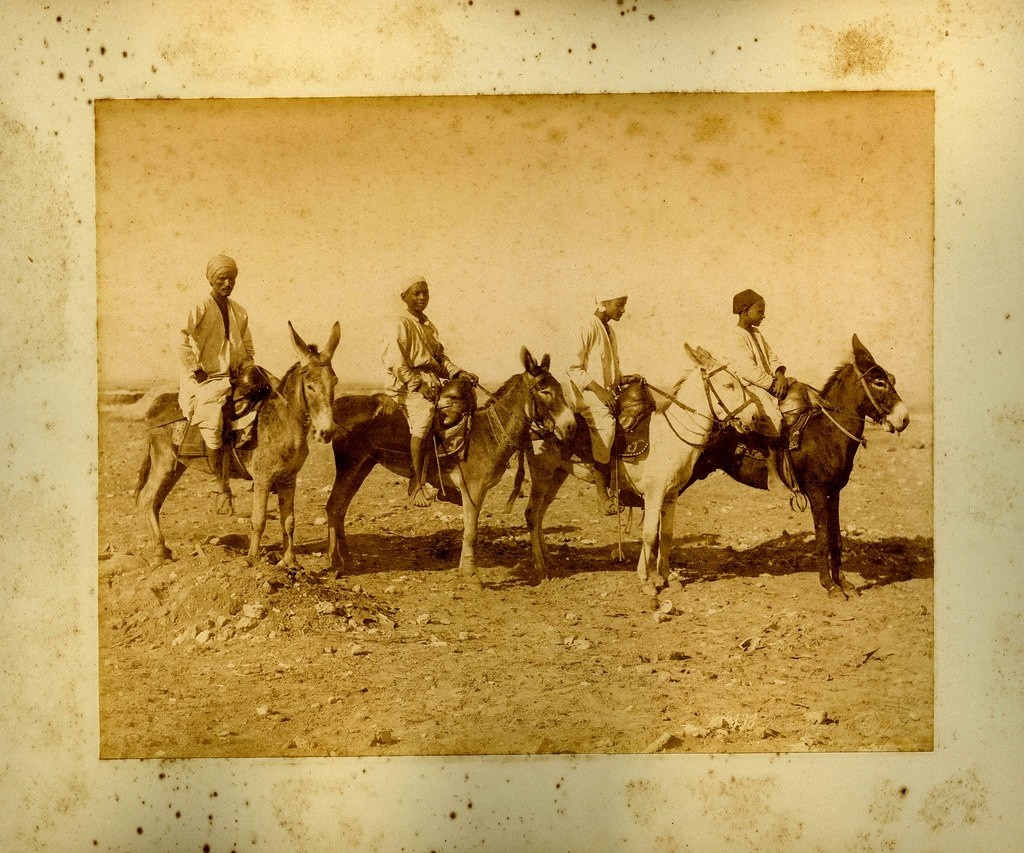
[710,288,797,500]
[374,275,478,507]
[176,253,255,517]
[563,288,641,516]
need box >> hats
[401,274,427,294]
[733,289,763,314]
[206,254,238,286]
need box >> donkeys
[131,320,342,573]
[324,343,580,589]
[652,334,911,602]
[522,341,762,596]
